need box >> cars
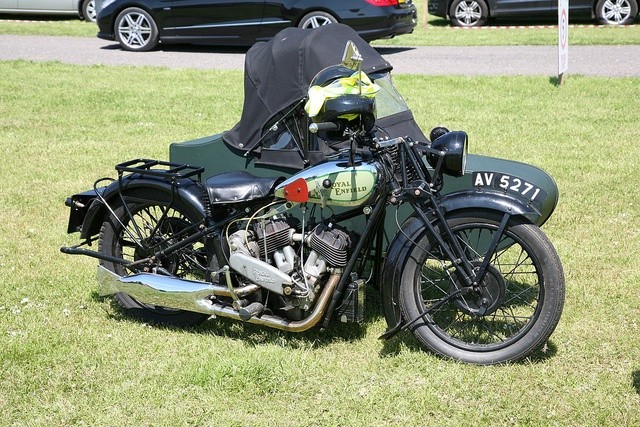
[96,0,416,50]
[427,0,634,24]
[0,0,112,23]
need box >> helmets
[308,65,376,139]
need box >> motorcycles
[61,120,564,366]
